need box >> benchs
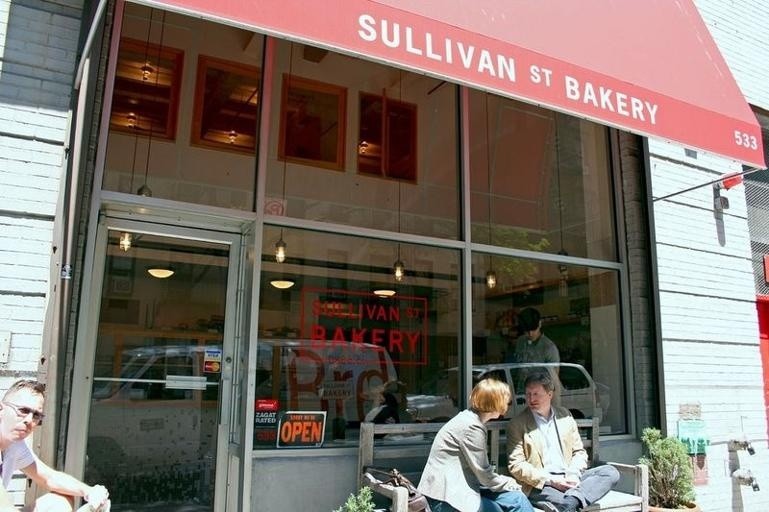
[357,416,650,512]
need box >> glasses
[3,401,45,423]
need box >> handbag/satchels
[387,469,432,512]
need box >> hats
[517,308,540,331]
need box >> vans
[384,362,603,435]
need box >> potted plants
[638,426,699,512]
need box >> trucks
[83,337,396,502]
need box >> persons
[0,379,112,512]
[512,306,562,400]
[365,379,419,441]
[416,377,535,511]
[505,372,621,511]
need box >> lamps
[137,11,173,214]
[146,262,176,283]
[477,90,503,291]
[386,71,415,285]
[265,40,305,269]
[369,285,400,300]
[119,10,158,255]
[270,277,296,294]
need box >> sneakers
[543,502,575,512]
